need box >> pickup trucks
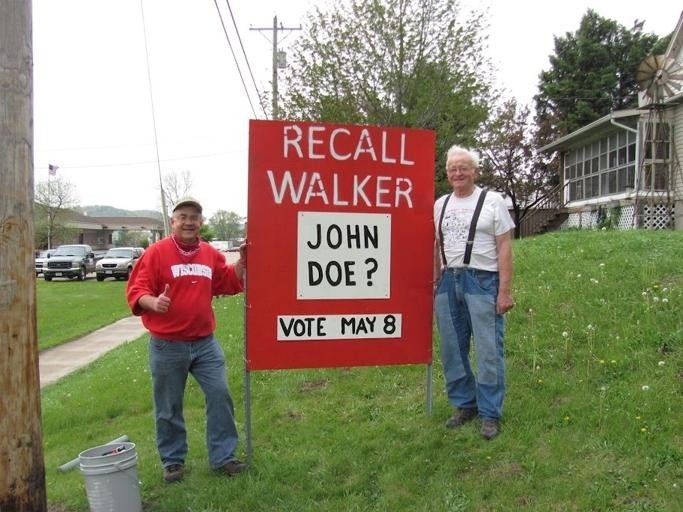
[43,244,108,283]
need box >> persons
[126,198,248,482]
[433,143,515,443]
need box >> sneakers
[163,464,184,482]
[219,460,248,477]
[446,407,478,428]
[480,418,499,440]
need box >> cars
[223,246,240,253]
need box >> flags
[48,163,59,176]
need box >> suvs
[96,248,140,280]
[37,249,53,274]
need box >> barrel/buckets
[78,441,142,512]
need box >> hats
[172,197,202,213]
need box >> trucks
[208,240,232,251]
